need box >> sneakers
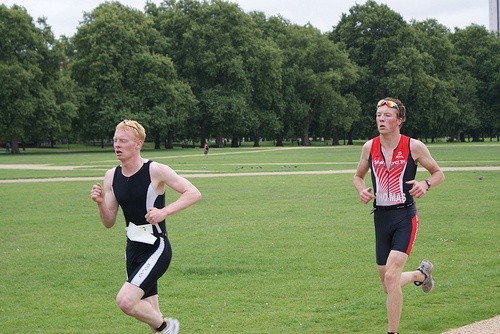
[414,259,434,294]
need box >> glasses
[377,100,402,117]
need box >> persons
[353,96,445,334]
[91,119,202,334]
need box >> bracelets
[425,180,431,191]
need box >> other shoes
[154,318,180,334]
